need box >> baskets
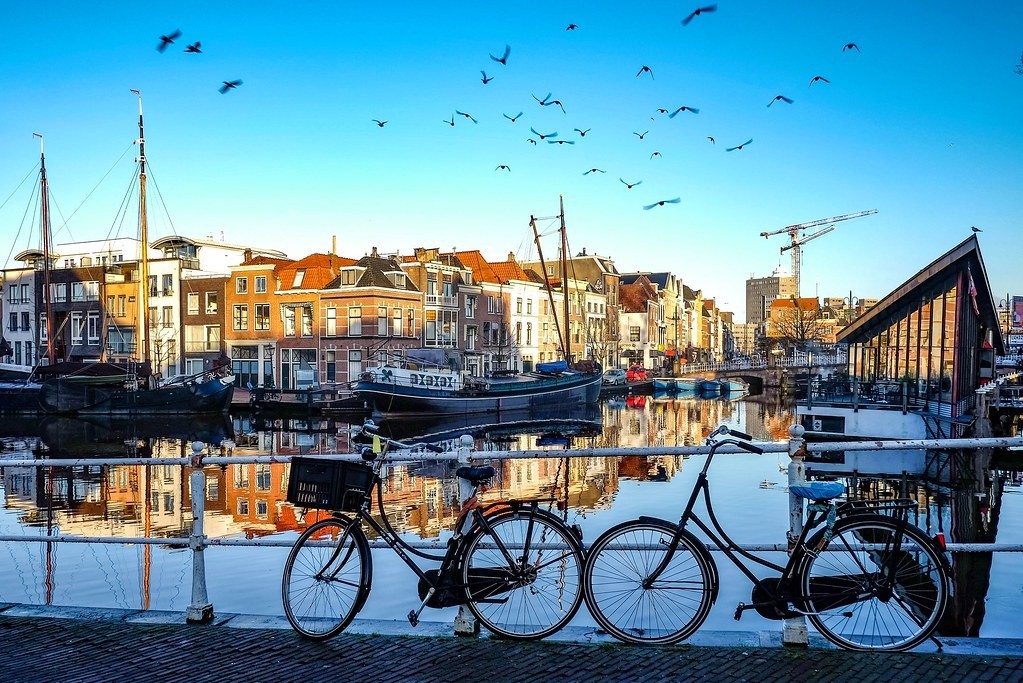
[287,457,374,512]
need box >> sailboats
[0,88,238,415]
[353,193,605,417]
[0,132,163,416]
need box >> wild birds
[970,225,984,234]
[155,4,860,212]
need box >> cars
[625,364,647,382]
[602,369,627,386]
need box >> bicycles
[581,426,959,653]
[281,419,587,642]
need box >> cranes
[760,208,879,298]
[780,224,835,298]
